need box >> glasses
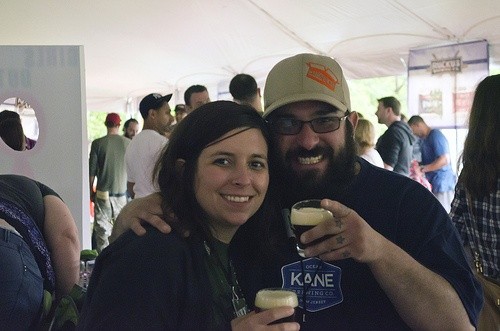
[268,112,353,136]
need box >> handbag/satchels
[410,159,432,193]
[474,271,500,331]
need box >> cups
[254,287,298,326]
[291,199,335,258]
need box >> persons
[400,113,407,123]
[444,73,499,329]
[352,119,384,168]
[79,100,310,331]
[375,96,415,178]
[87,81,213,265]
[408,115,454,214]
[230,73,265,117]
[1,172,79,330]
[107,53,499,331]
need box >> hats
[106,113,122,124]
[139,93,174,118]
[174,104,186,112]
[261,52,349,119]
